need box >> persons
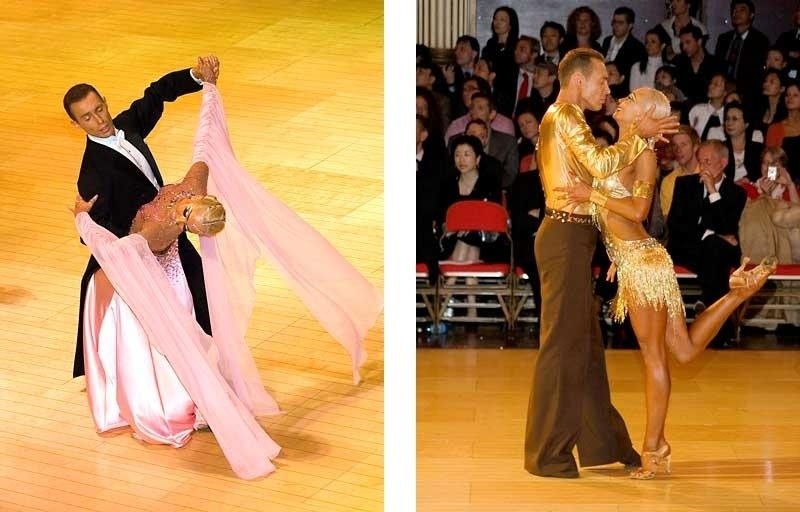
[416,0,800,351]
[63,57,220,377]
[551,87,781,481]
[67,57,384,481]
[522,46,681,481]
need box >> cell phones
[767,165,778,181]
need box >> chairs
[416,190,800,349]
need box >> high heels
[727,253,782,289]
[631,444,675,480]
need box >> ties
[546,55,553,62]
[729,32,744,75]
[518,72,529,100]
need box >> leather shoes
[694,300,705,314]
[614,444,641,465]
